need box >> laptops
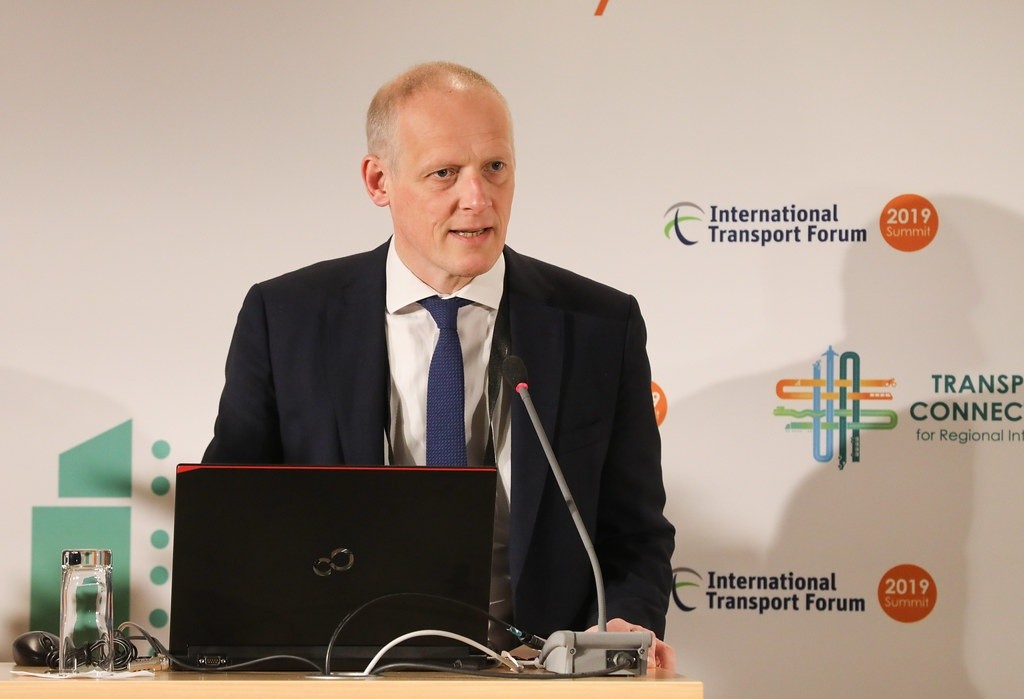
[13,631,60,666]
[169,463,499,671]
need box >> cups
[58,549,115,676]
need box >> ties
[416,295,475,468]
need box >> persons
[202,61,676,671]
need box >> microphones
[501,354,653,677]
